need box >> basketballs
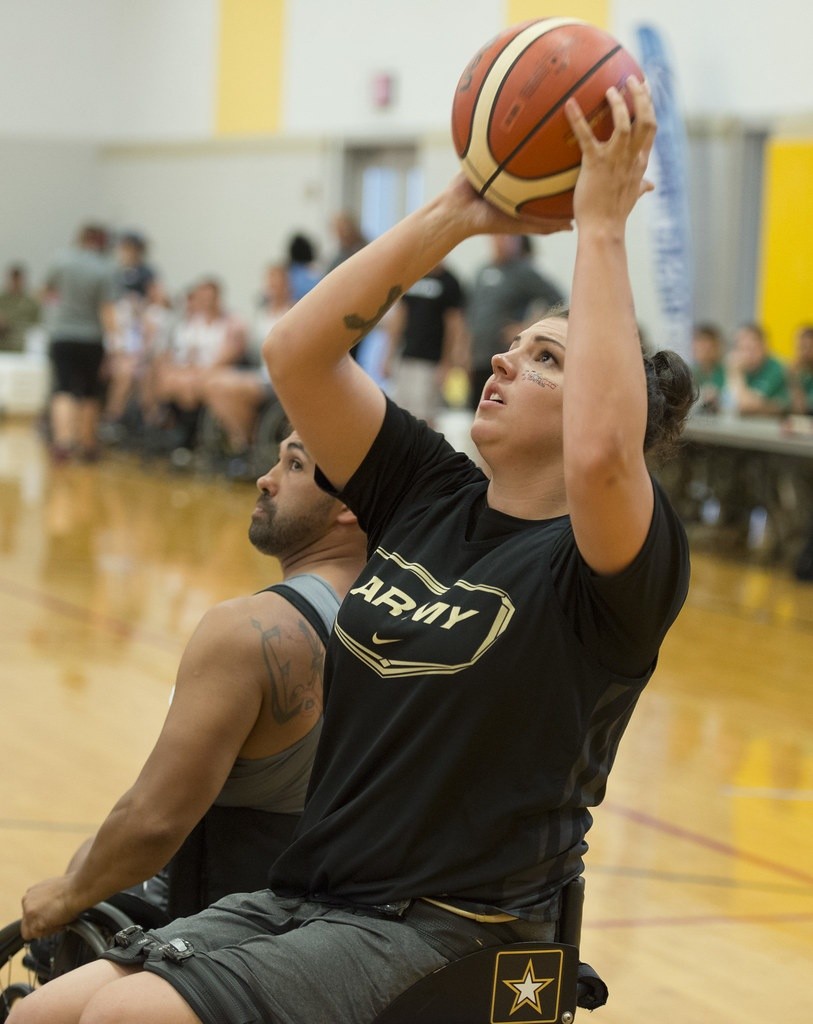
[451,17,652,224]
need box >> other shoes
[53,445,69,460]
[84,447,100,463]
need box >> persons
[0,207,813,589]
[1,76,696,1024]
[0,428,373,938]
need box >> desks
[679,414,813,562]
